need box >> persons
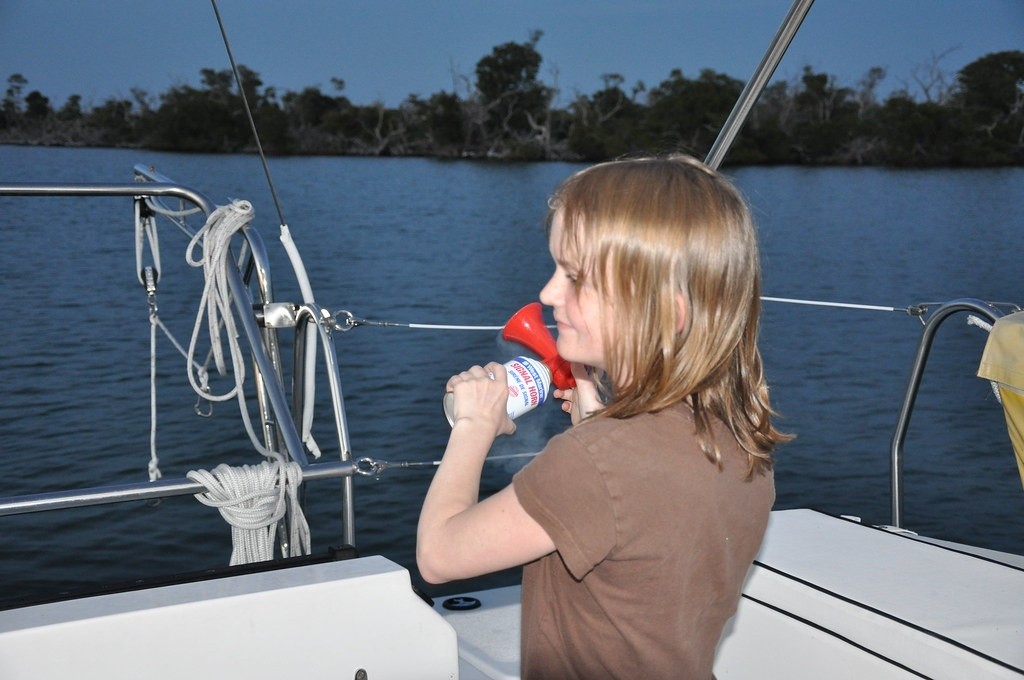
[416,154,797,680]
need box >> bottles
[442,356,554,429]
